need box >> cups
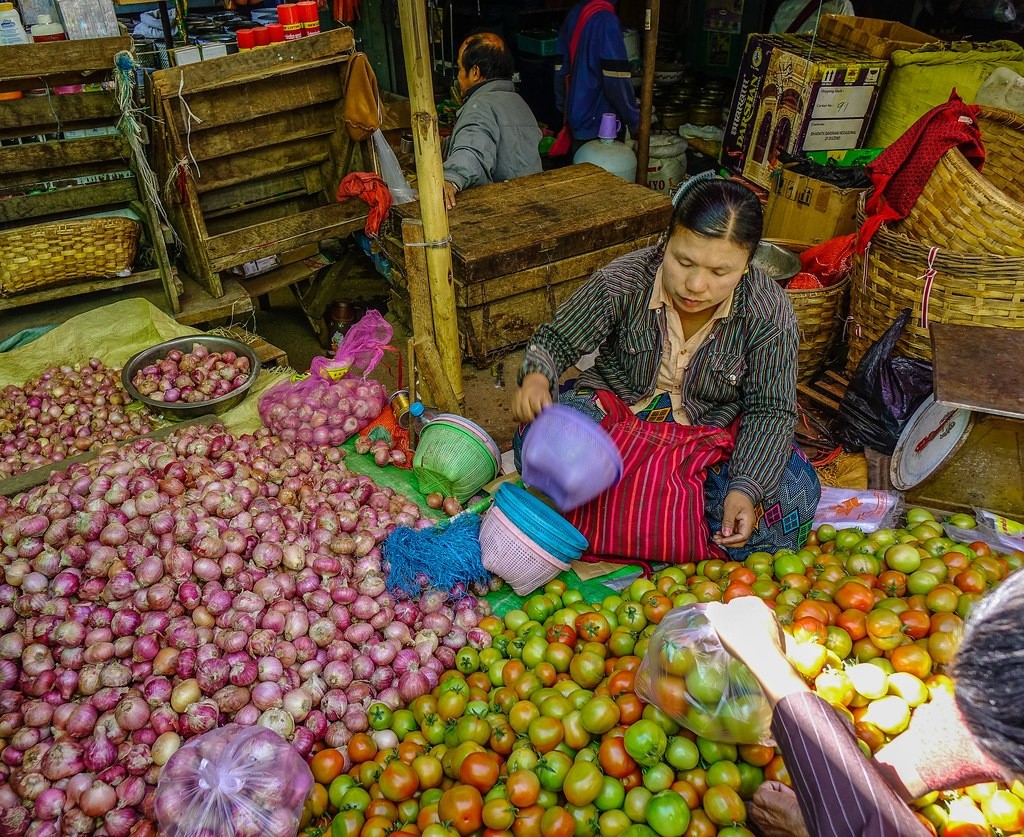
[598,113,620,138]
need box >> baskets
[842,186,1024,386]
[414,406,625,596]
[754,236,857,383]
[0,215,142,298]
[894,105,1024,256]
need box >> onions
[0,343,502,837]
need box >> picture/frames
[707,30,731,67]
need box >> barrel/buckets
[573,137,637,184]
[635,77,736,130]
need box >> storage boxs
[816,10,950,64]
[167,41,228,67]
[382,161,675,360]
[757,164,867,245]
[718,28,891,194]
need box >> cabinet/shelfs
[1,22,181,319]
[145,17,386,298]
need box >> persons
[550,0,639,165]
[510,176,820,563]
[767,0,855,35]
[413,32,542,211]
[697,567,1023,837]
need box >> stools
[206,324,289,368]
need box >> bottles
[410,401,439,438]
[0,2,30,46]
[277,3,303,42]
[30,15,65,44]
[296,1,321,37]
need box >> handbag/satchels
[256,310,394,447]
[155,725,317,836]
[635,599,793,747]
[833,309,936,458]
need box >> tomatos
[295,508,1024,837]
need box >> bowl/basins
[653,62,686,82]
[121,334,260,423]
